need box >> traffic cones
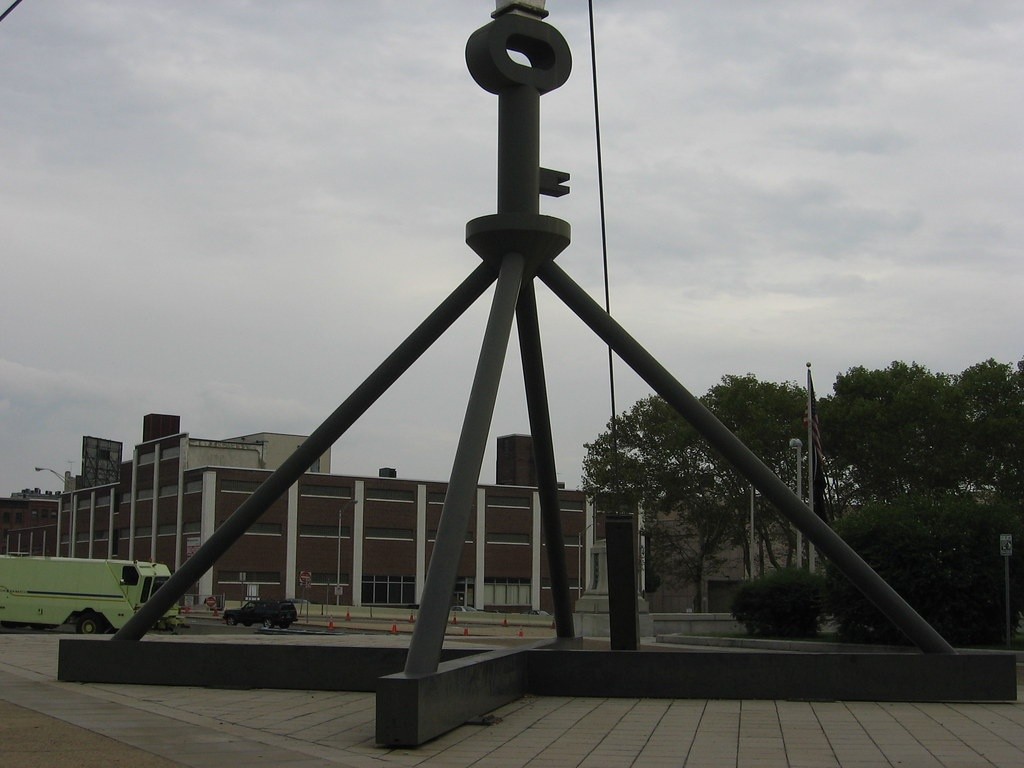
[463,621,469,636]
[391,620,398,635]
[408,609,415,622]
[185,601,193,614]
[451,612,457,624]
[517,625,525,637]
[502,615,509,627]
[327,614,336,631]
[345,608,351,621]
[212,606,220,616]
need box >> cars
[525,610,550,616]
[452,606,477,612]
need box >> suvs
[223,600,298,629]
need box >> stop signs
[207,597,216,606]
[300,571,311,585]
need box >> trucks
[0,552,180,634]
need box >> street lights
[35,467,74,557]
[579,524,592,600]
[336,500,358,606]
[789,438,803,571]
